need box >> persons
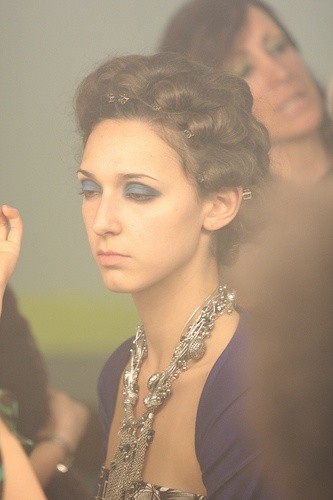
[0,285,92,500]
[153,0,333,303]
[1,52,272,500]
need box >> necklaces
[95,283,237,500]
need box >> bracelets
[38,432,74,478]
